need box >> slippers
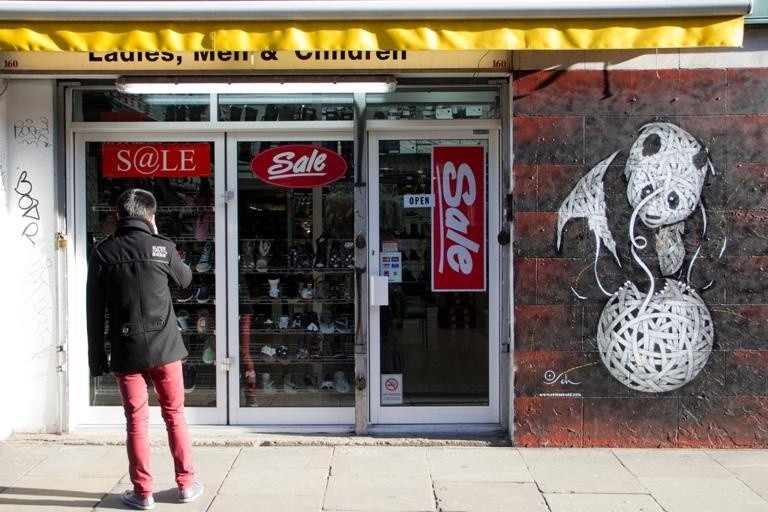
[262,373,278,397]
[283,374,298,397]
[260,344,277,364]
[334,316,352,334]
[319,310,335,334]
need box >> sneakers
[238,275,355,300]
[251,313,302,330]
[177,244,193,269]
[246,398,260,407]
[196,309,211,335]
[196,282,212,304]
[334,372,351,395]
[120,489,155,510]
[195,241,215,274]
[302,310,320,333]
[86,176,214,242]
[177,285,196,302]
[202,337,216,364]
[178,480,204,503]
[320,375,335,393]
[182,362,198,394]
[277,343,289,360]
[238,234,355,273]
[380,172,431,282]
[296,337,348,364]
[175,310,190,332]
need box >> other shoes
[164,104,482,120]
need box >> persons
[89,187,201,508]
[206,239,260,407]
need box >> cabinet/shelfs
[90,203,355,407]
